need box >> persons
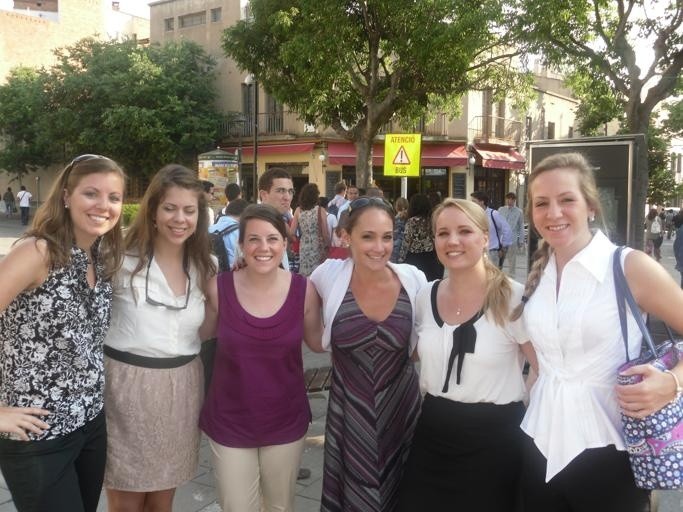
[0,153,124,512]
[194,179,682,401]
[412,198,537,511]
[102,166,217,511]
[198,204,330,511]
[234,168,311,479]
[516,152,682,511]
[3,187,14,219]
[17,186,31,225]
[306,197,428,511]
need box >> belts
[490,249,498,251]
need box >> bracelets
[665,368,681,405]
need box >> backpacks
[208,221,241,274]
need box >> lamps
[467,153,476,175]
[319,151,326,173]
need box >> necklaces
[446,283,478,317]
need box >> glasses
[346,195,393,220]
[143,264,192,312]
[64,153,110,188]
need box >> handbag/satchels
[12,200,17,213]
[318,251,331,264]
[650,216,661,234]
[610,241,683,492]
[499,244,504,266]
